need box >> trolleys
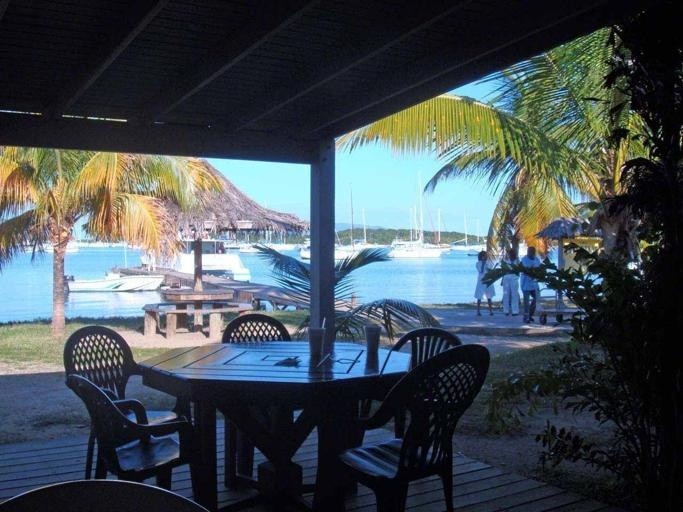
[531,278,589,325]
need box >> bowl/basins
[170,283,181,288]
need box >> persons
[519,245,542,323]
[500,250,521,316]
[474,250,498,317]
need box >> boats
[42,241,81,255]
[238,242,297,254]
[69,274,165,291]
[18,240,42,254]
[157,236,251,282]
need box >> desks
[160,288,236,336]
[136,339,414,512]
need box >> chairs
[62,366,200,496]
[329,340,491,511]
[364,324,465,444]
[219,308,296,482]
[61,325,183,481]
[1,474,216,512]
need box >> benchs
[155,305,245,336]
[138,300,254,342]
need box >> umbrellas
[78,158,309,336]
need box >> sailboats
[296,176,527,262]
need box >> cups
[307,326,326,357]
[365,324,382,354]
